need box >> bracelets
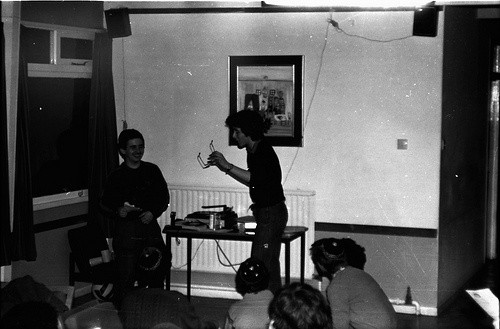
[226,164,233,174]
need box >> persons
[223,237,397,329]
[98,129,171,308]
[0,299,58,329]
[207,109,288,292]
[247,87,288,119]
[285,120,287,120]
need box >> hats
[240,260,265,284]
[319,240,344,263]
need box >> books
[182,221,207,231]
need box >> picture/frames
[227,54,303,146]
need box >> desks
[163,224,308,303]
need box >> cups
[233,220,246,233]
[209,213,221,230]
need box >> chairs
[68,224,115,286]
